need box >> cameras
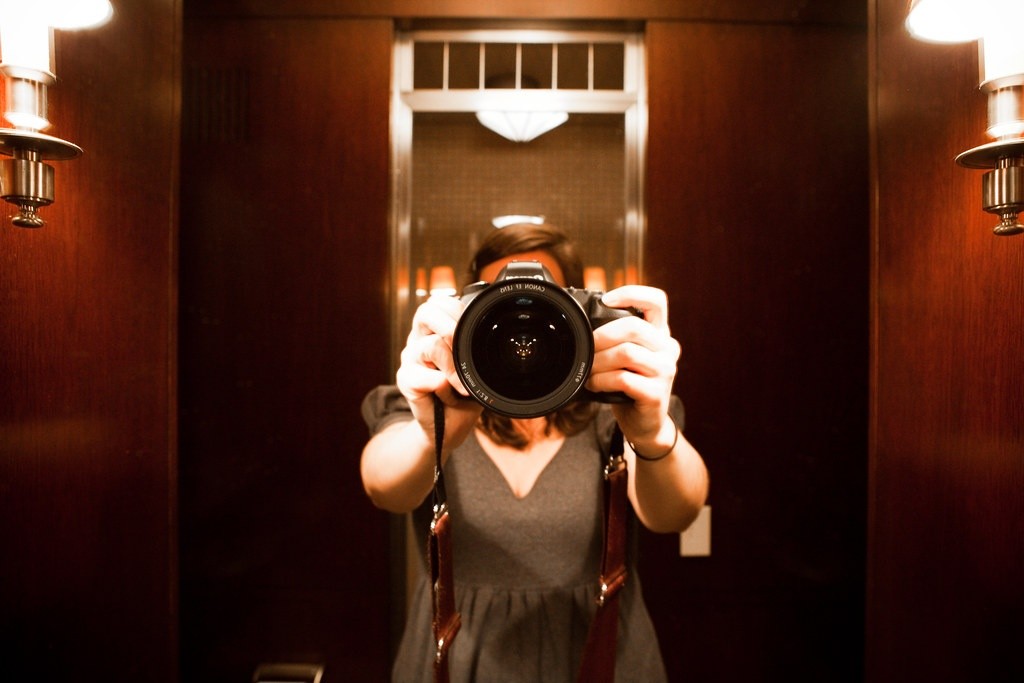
[454,260,643,419]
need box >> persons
[359,220,707,683]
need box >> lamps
[900,0,1024,234]
[478,72,568,147]
[0,0,112,226]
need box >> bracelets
[627,412,678,461]
